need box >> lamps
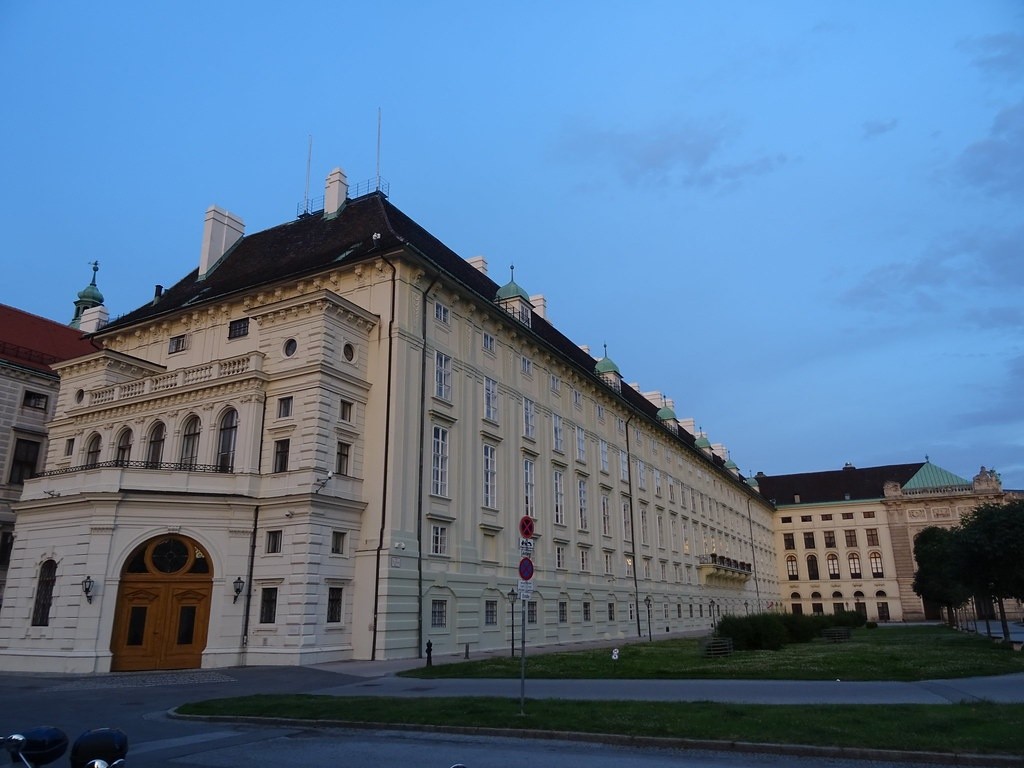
[82,575,94,605]
[233,577,245,604]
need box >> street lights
[507,588,519,657]
[744,602,748,617]
[644,596,651,642]
[710,600,718,640]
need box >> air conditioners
[699,553,752,572]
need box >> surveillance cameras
[401,546,405,550]
[43,490,54,493]
[328,470,334,479]
[285,513,291,516]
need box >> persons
[979,466,991,481]
